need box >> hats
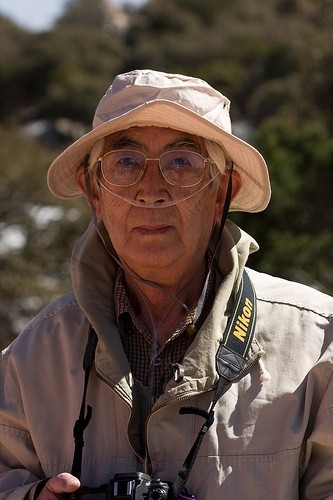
[49,70,271,216]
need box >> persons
[2,69,332,500]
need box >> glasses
[92,146,204,187]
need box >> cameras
[63,471,196,500]
